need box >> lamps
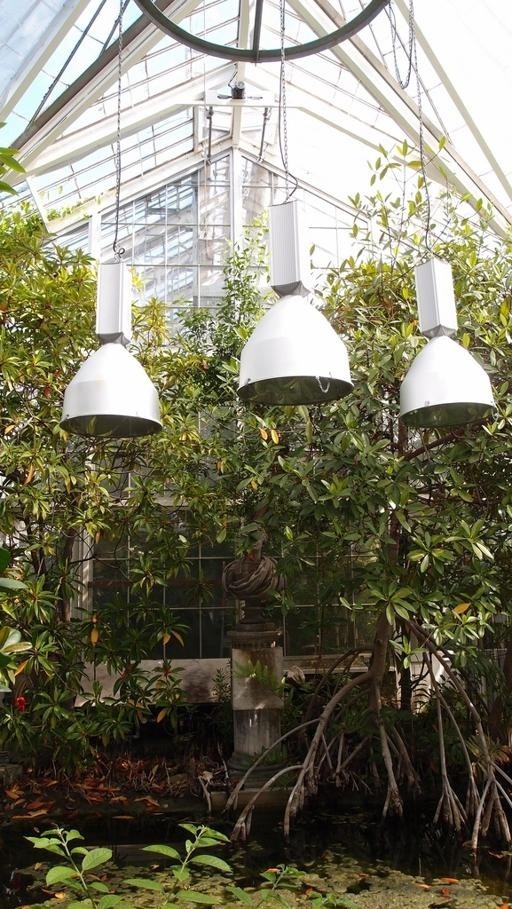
[397,0,497,424]
[59,1,163,437]
[236,1,354,404]
